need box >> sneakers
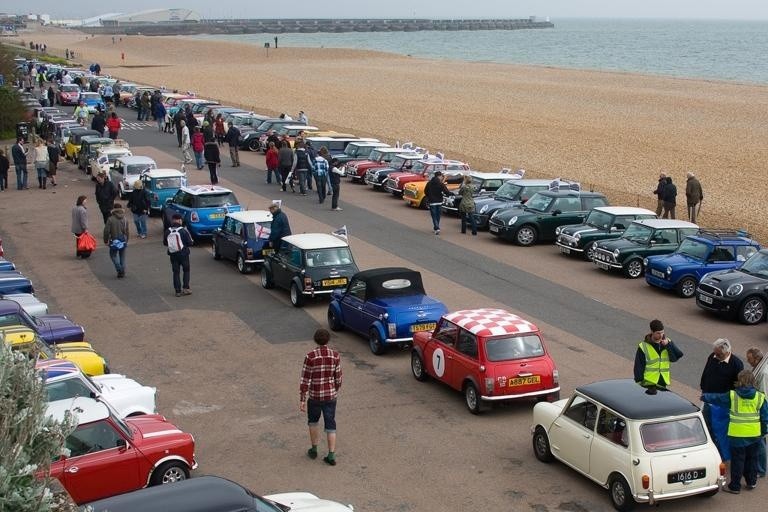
[324,457,336,465]
[183,288,192,295]
[309,449,317,458]
[722,485,740,494]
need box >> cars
[18,355,160,430]
[327,264,450,354]
[19,473,357,512]
[137,167,184,217]
[5,397,197,507]
[259,231,362,309]
[214,114,521,217]
[109,155,158,200]
[408,308,561,415]
[208,210,276,275]
[591,218,701,278]
[556,206,658,259]
[0,57,242,181]
[0,238,112,382]
[162,184,242,247]
[529,377,726,510]
[492,188,610,246]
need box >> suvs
[696,248,768,324]
[643,229,761,299]
[465,177,570,232]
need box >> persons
[425,171,478,235]
[29,41,47,51]
[700,368,767,496]
[1,135,59,190]
[135,91,242,185]
[162,213,194,297]
[686,172,703,223]
[122,53,125,60]
[71,174,151,279]
[698,336,745,464]
[39,86,55,107]
[280,113,292,120]
[66,48,75,59]
[268,203,294,252]
[742,345,768,478]
[298,111,308,124]
[632,319,686,388]
[266,130,344,211]
[18,62,101,93]
[72,101,121,140]
[645,388,658,395]
[298,328,343,467]
[654,172,676,220]
[103,80,121,106]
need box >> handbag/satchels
[109,237,127,251]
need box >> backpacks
[166,227,184,253]
